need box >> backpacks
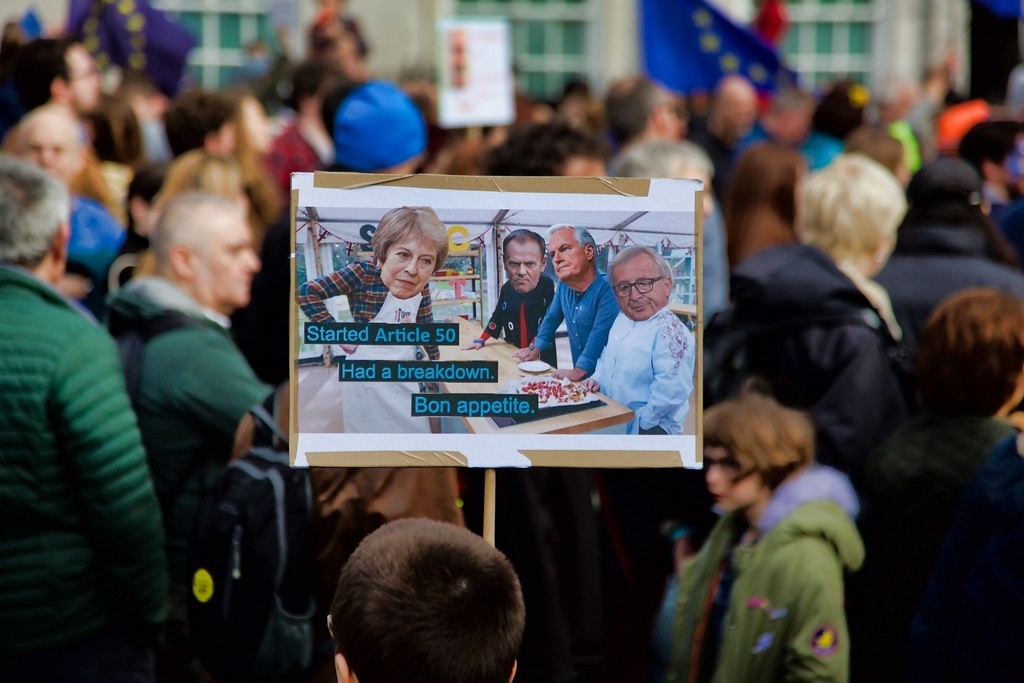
[175,392,343,682]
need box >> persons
[668,390,865,683]
[0,0,1024,683]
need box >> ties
[519,300,527,349]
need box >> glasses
[613,275,667,298]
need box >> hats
[334,81,426,173]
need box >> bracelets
[529,344,534,350]
[473,339,485,347]
